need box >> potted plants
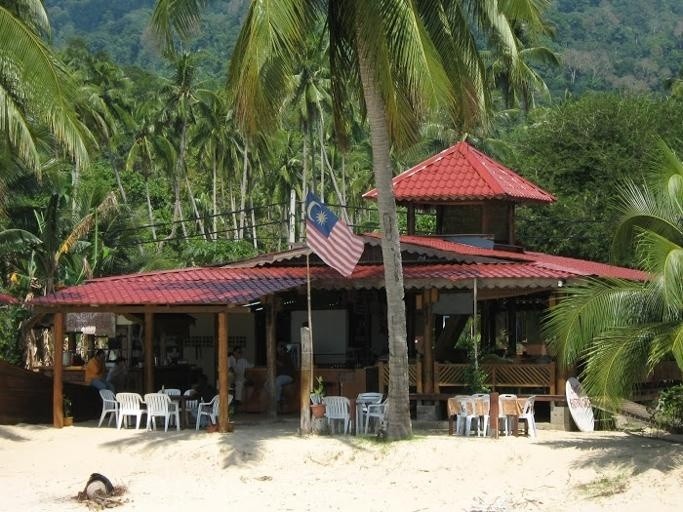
[63,395,74,426]
[310,375,327,418]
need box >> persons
[190,350,217,392]
[167,350,193,385]
[226,345,254,406]
[275,339,297,402]
[106,357,129,394]
[86,348,115,398]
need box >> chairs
[446,393,537,437]
[324,392,390,435]
[97,388,233,432]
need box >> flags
[304,189,365,280]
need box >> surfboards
[565,377,595,433]
